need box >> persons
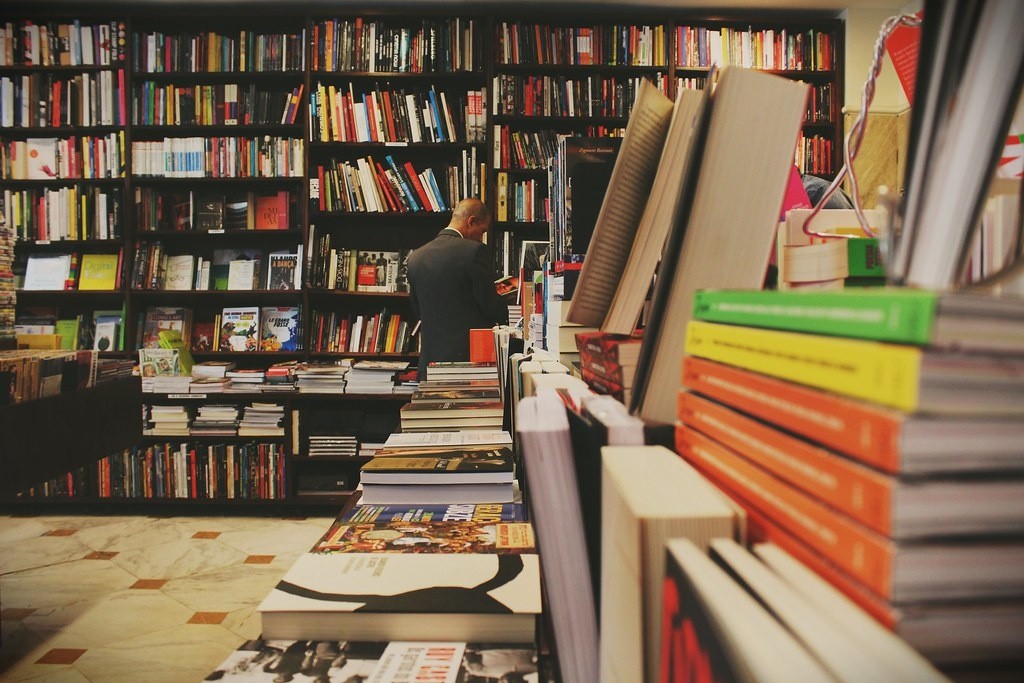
[406,197,509,382]
[360,252,388,285]
[495,276,519,295]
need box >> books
[1,17,838,501]
[200,0,1024,683]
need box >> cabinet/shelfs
[308,6,489,357]
[5,362,418,515]
[489,8,671,305]
[0,9,129,356]
[671,14,844,192]
[129,9,308,356]
